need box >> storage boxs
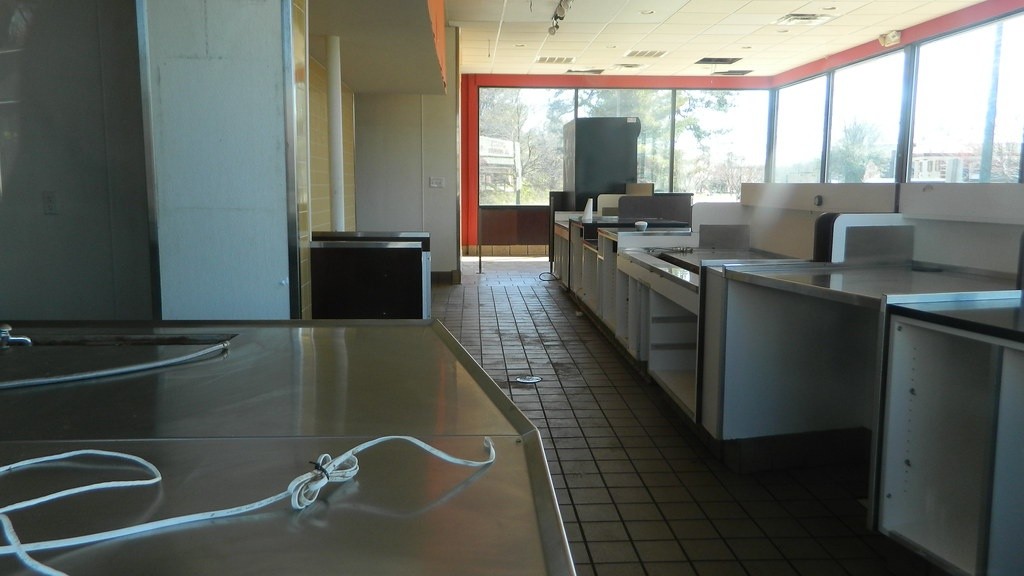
[309,231,430,319]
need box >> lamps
[771,13,832,29]
[548,0,574,35]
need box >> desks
[721,183,1024,473]
[876,296,1024,576]
[617,183,901,435]
[0,319,578,576]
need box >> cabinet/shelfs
[554,183,655,292]
[567,193,694,351]
[309,0,447,96]
